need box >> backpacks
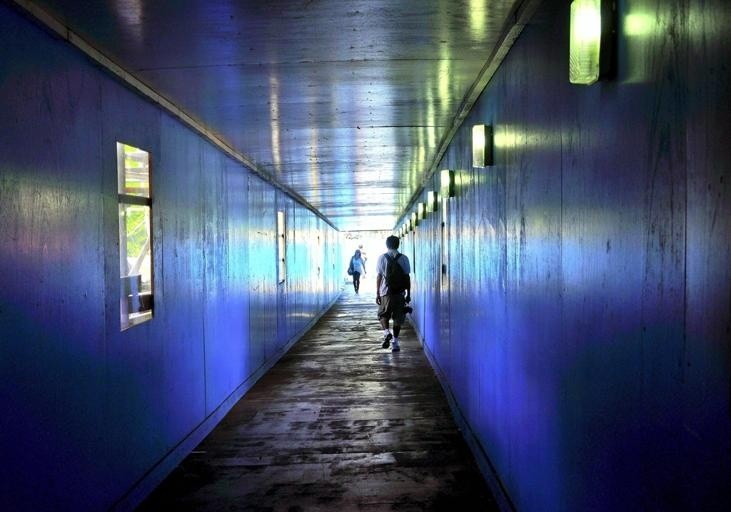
[382,254,408,294]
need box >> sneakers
[392,342,400,351]
[382,331,392,348]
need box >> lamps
[397,191,438,240]
[472,124,493,168]
[568,0,615,87]
[441,170,454,198]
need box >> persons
[376,235,410,350]
[349,245,367,295]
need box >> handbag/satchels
[348,264,354,275]
